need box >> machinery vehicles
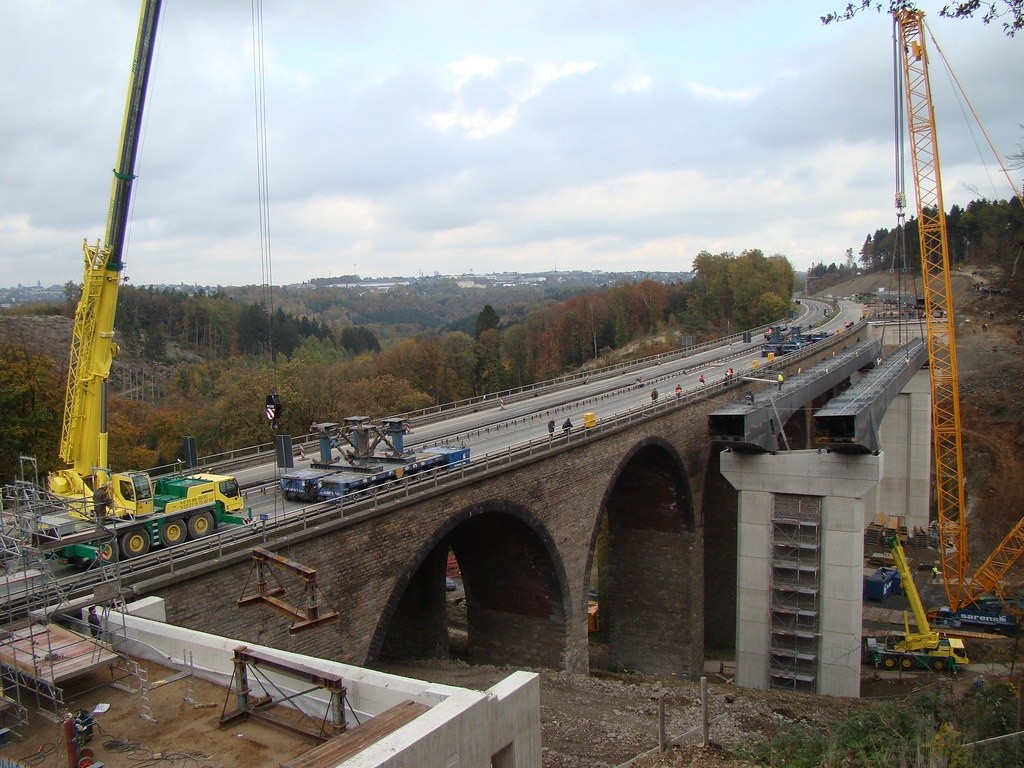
[865,533,970,669]
[876,5,1024,642]
[23,0,288,571]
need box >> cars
[446,577,456,591]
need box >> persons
[562,418,573,433]
[982,323,988,331]
[548,417,555,436]
[698,375,705,384]
[777,372,783,392]
[724,367,733,376]
[932,565,937,579]
[651,388,658,403]
[124,490,133,500]
[87,606,103,640]
[675,384,683,396]
[973,283,979,290]
[93,484,113,532]
[971,271,977,277]
